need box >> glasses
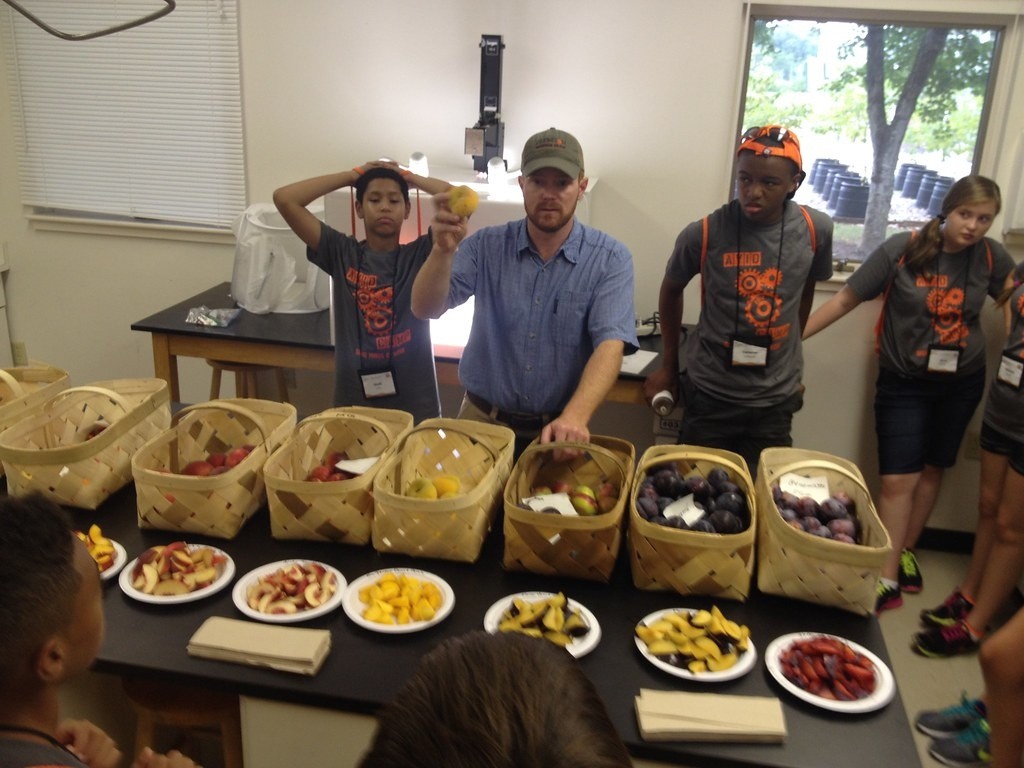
[739,126,802,154]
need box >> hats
[520,126,584,179]
[736,125,803,174]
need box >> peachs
[405,474,466,499]
[778,634,876,701]
[133,541,227,595]
[445,185,479,216]
[302,451,357,482]
[74,526,116,573]
[155,444,256,524]
[85,425,106,440]
[246,563,335,613]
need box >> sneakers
[897,548,924,592]
[875,580,902,617]
[911,619,990,660]
[920,586,979,629]
[915,695,989,739]
[929,716,995,764]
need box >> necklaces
[0,724,80,761]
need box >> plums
[515,482,619,516]
[773,486,861,545]
[497,592,591,647]
[634,604,748,672]
[636,454,750,535]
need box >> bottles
[651,389,674,415]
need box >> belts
[467,389,563,430]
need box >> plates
[634,608,758,682]
[483,592,602,659]
[101,538,128,581]
[342,568,455,634]
[764,630,896,714]
[232,558,348,623]
[118,544,236,604]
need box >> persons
[915,605,1024,768]
[360,630,633,768]
[642,125,833,483]
[272,160,453,431]
[0,490,194,768]
[802,174,1017,616]
[411,128,640,461]
[911,260,1024,658]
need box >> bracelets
[399,170,411,177]
[352,167,365,175]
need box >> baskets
[131,397,296,538]
[629,443,755,601]
[262,407,414,544]
[754,446,891,616]
[0,379,171,511]
[502,432,635,582]
[0,366,70,478]
[372,417,514,564]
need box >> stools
[120,673,243,768]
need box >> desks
[0,419,924,768]
[131,279,700,468]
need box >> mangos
[359,573,443,625]
